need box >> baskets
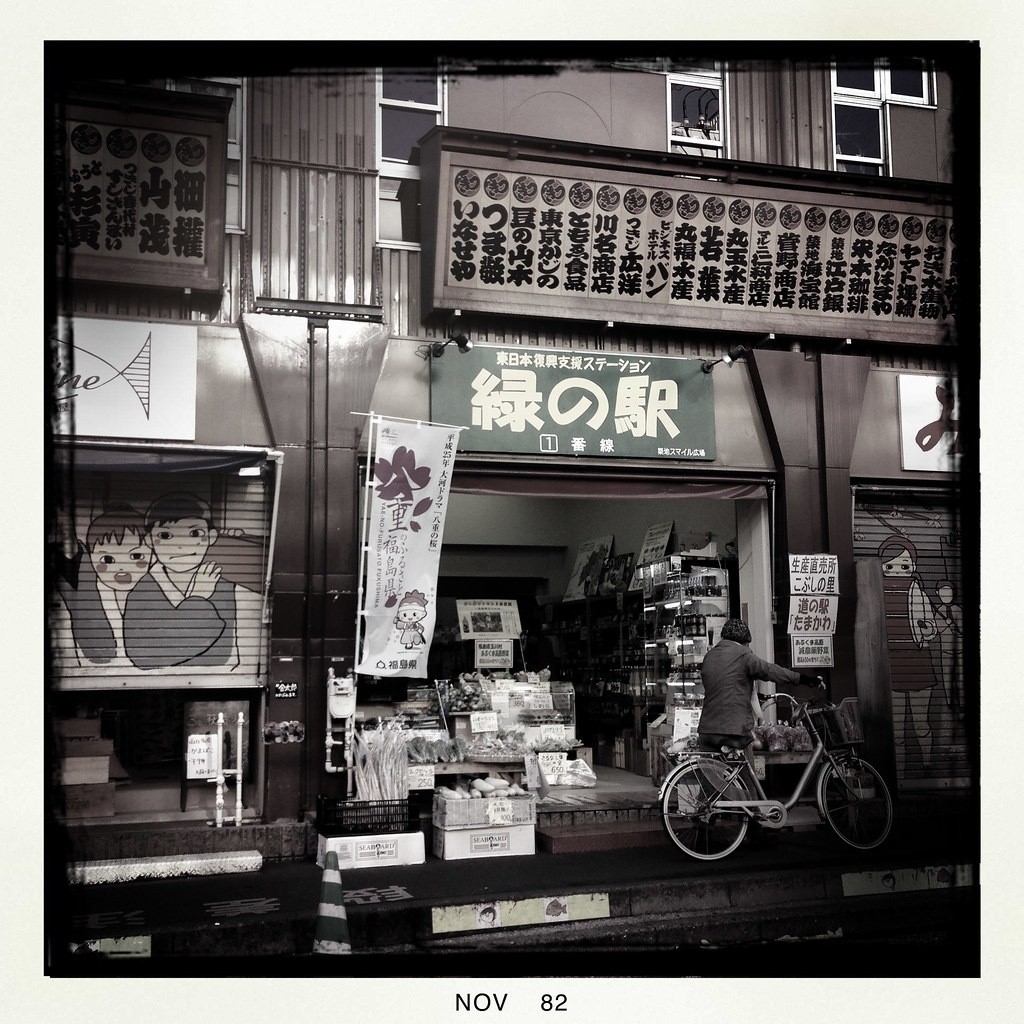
[801,696,866,748]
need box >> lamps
[432,329,475,357]
[702,344,748,374]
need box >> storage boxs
[665,705,703,742]
[52,719,116,820]
[315,789,536,870]
[592,735,652,777]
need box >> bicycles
[646,691,895,863]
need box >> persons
[695,618,826,854]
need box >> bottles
[607,664,701,697]
[661,613,725,654]
[664,576,721,600]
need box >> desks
[657,745,860,828]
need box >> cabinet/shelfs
[538,588,679,745]
[637,552,741,728]
